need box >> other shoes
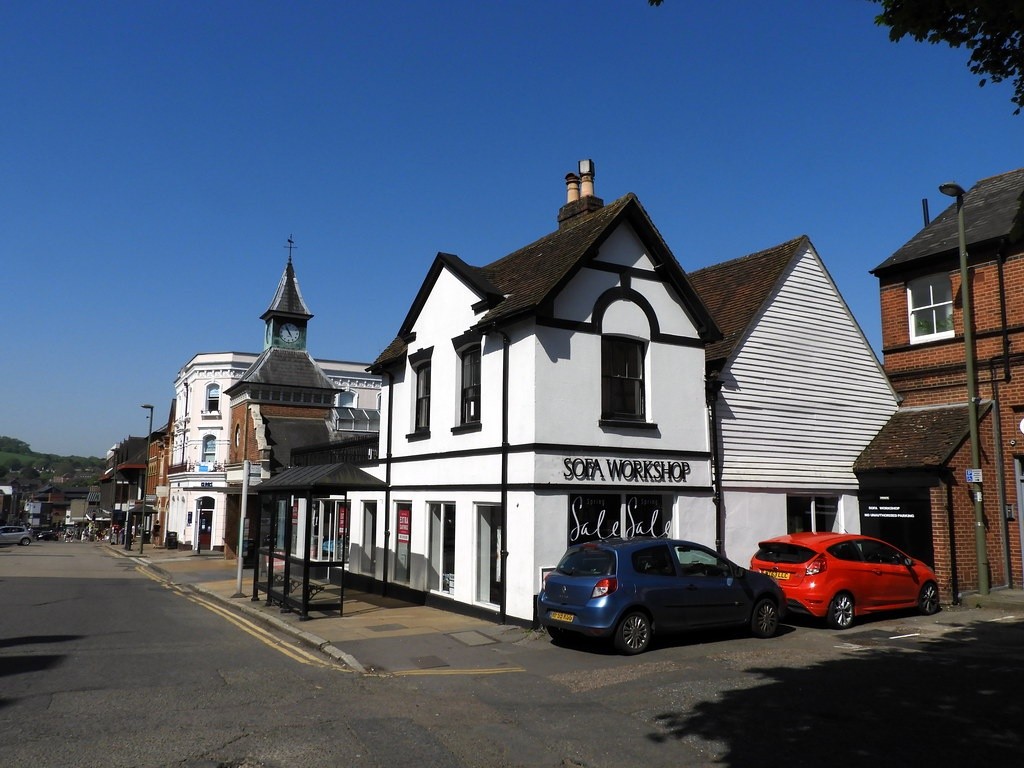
[153,545,158,548]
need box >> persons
[64,529,74,542]
[119,526,126,545]
[151,520,161,549]
[83,526,109,542]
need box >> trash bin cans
[142,530,151,544]
[166,531,178,549]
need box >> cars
[749,531,940,630]
[536,538,786,656]
[36,531,59,541]
[0,526,32,546]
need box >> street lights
[138,404,154,555]
